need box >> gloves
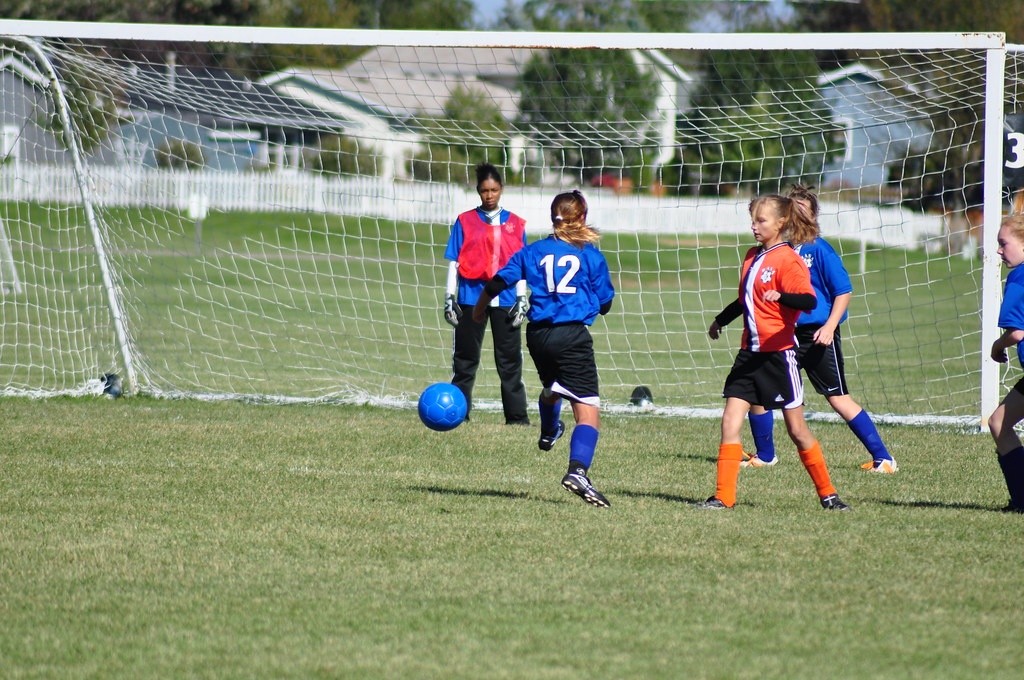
[444,297,462,327]
[508,297,529,327]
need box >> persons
[473,190,616,507]
[697,195,849,510]
[444,163,531,425]
[988,215,1024,513]
[741,182,901,474]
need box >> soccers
[418,383,467,432]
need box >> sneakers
[538,419,566,451]
[696,496,734,510]
[739,448,778,469]
[561,473,611,509]
[863,455,898,475]
[820,494,849,510]
